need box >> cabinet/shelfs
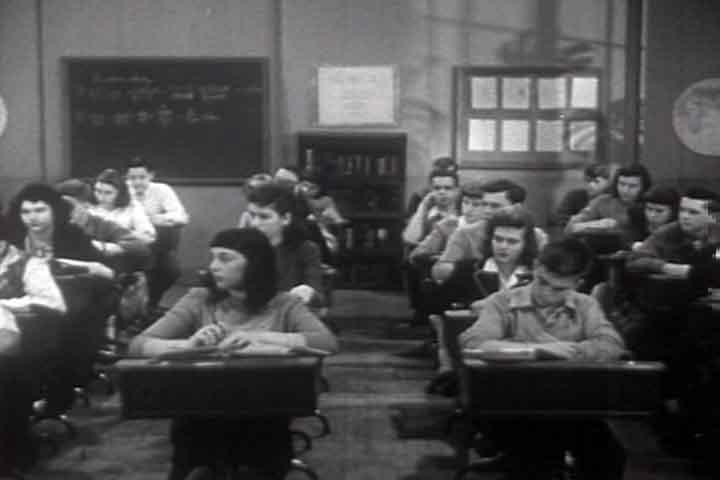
[293,126,409,293]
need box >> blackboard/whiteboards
[59,56,271,186]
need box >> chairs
[1,170,720,480]
